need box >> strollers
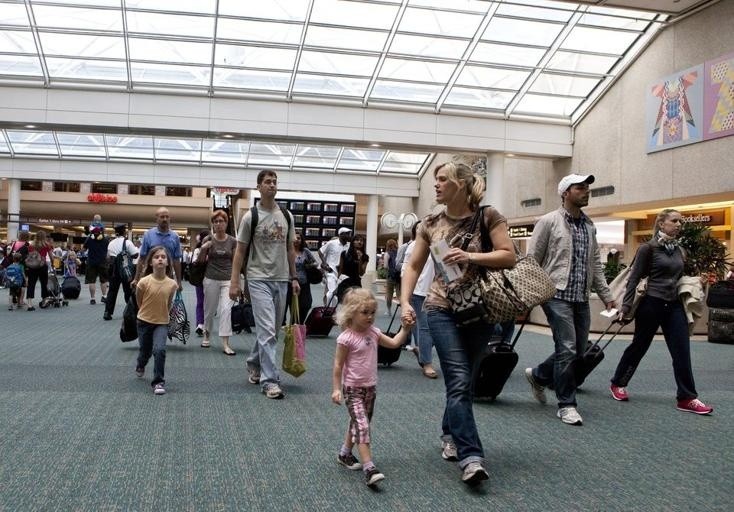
[38,265,70,308]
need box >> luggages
[62,275,81,300]
[548,339,605,390]
[469,336,519,401]
[376,330,403,368]
[305,306,337,338]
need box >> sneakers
[136,365,145,377]
[609,381,628,401]
[152,383,167,395]
[337,453,363,471]
[89,296,107,305]
[364,466,385,487]
[195,324,204,336]
[402,345,423,370]
[676,397,714,416]
[461,461,489,484]
[423,366,439,378]
[440,439,461,462]
[261,383,284,399]
[7,298,35,311]
[246,361,260,384]
[103,311,112,321]
[525,368,547,403]
[555,406,584,426]
[382,311,393,319]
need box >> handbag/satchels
[480,254,556,328]
[231,302,254,335]
[607,264,650,326]
[116,236,135,283]
[165,289,191,344]
[307,266,323,284]
[120,289,138,342]
[281,293,306,377]
[188,260,208,287]
[446,283,487,326]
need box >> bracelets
[290,277,299,280]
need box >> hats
[337,226,353,235]
[557,173,595,197]
[114,222,128,232]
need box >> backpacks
[26,246,45,269]
[47,273,61,296]
[1,265,26,288]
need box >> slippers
[224,348,236,355]
[201,340,210,348]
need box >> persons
[610,210,712,416]
[131,208,238,393]
[0,214,140,321]
[230,170,302,399]
[292,220,438,379]
[526,174,616,425]
[332,289,413,485]
[402,160,517,483]
